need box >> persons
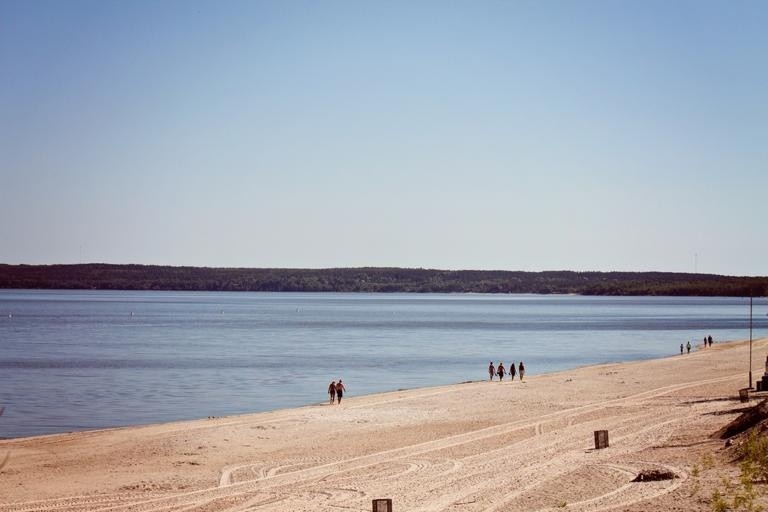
[328,382,336,404]
[489,362,495,381]
[498,362,506,381]
[680,344,683,355]
[510,363,516,381]
[686,341,691,353]
[708,335,712,347]
[336,380,346,404]
[704,337,707,345]
[519,362,525,380]
